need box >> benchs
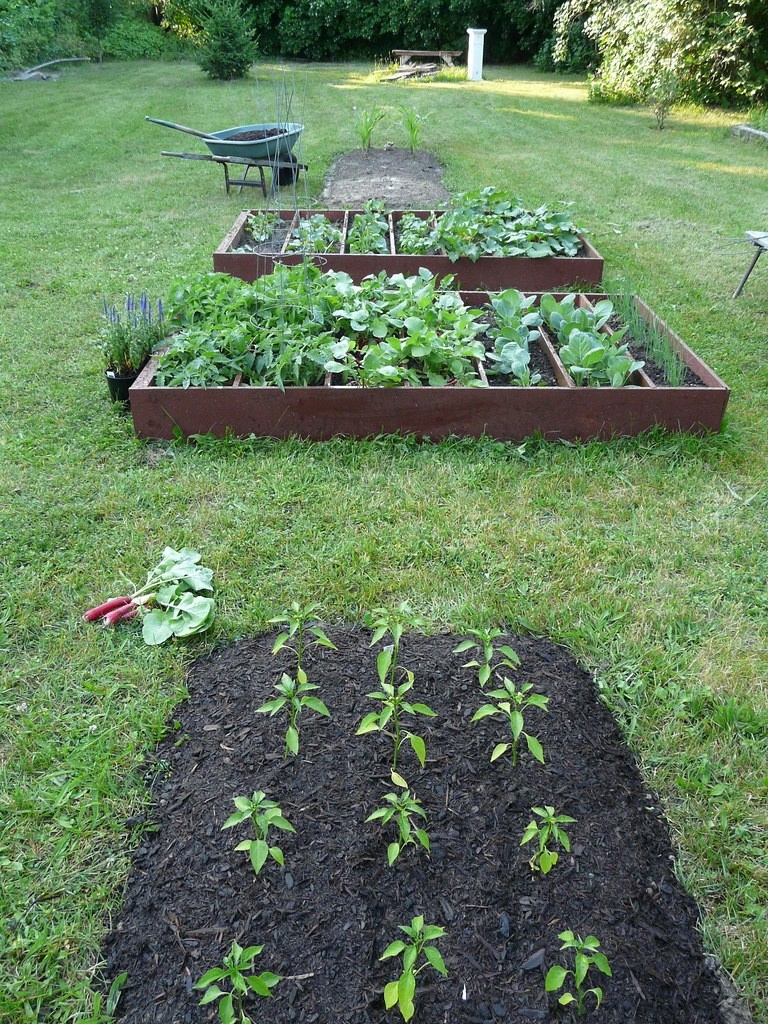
[393,49,463,68]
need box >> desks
[732,230,768,298]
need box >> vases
[105,368,138,409]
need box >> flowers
[100,291,167,375]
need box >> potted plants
[213,186,605,289]
[128,292,734,438]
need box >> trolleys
[145,115,309,197]
